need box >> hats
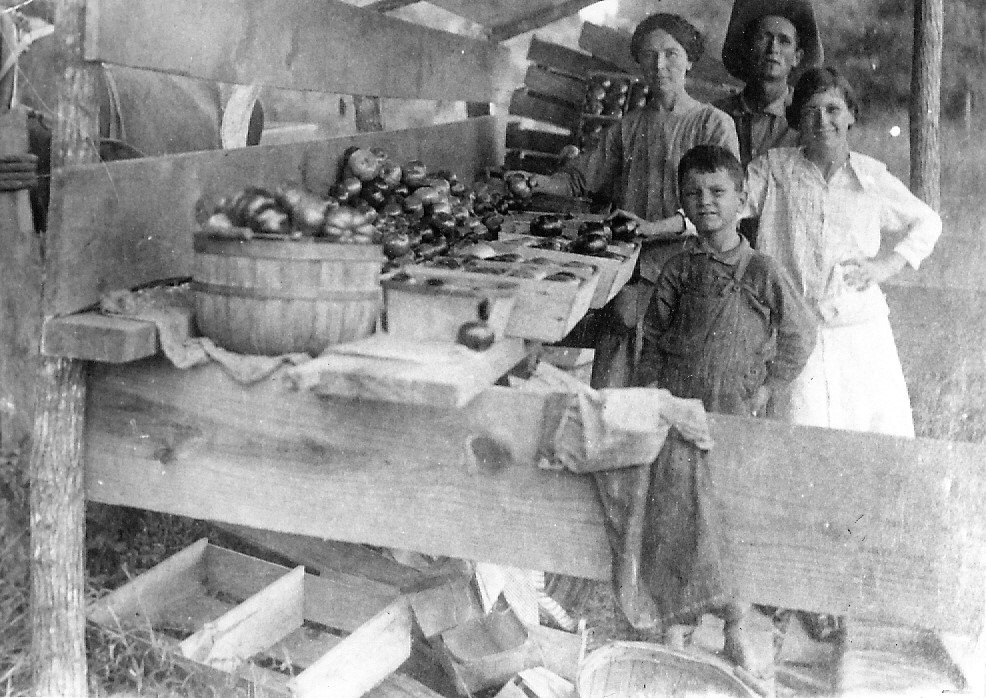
[722,0,826,83]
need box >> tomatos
[196,147,639,351]
[580,75,649,145]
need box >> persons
[639,142,818,682]
[498,12,738,535]
[704,0,830,252]
[605,66,941,644]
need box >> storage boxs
[380,233,643,342]
